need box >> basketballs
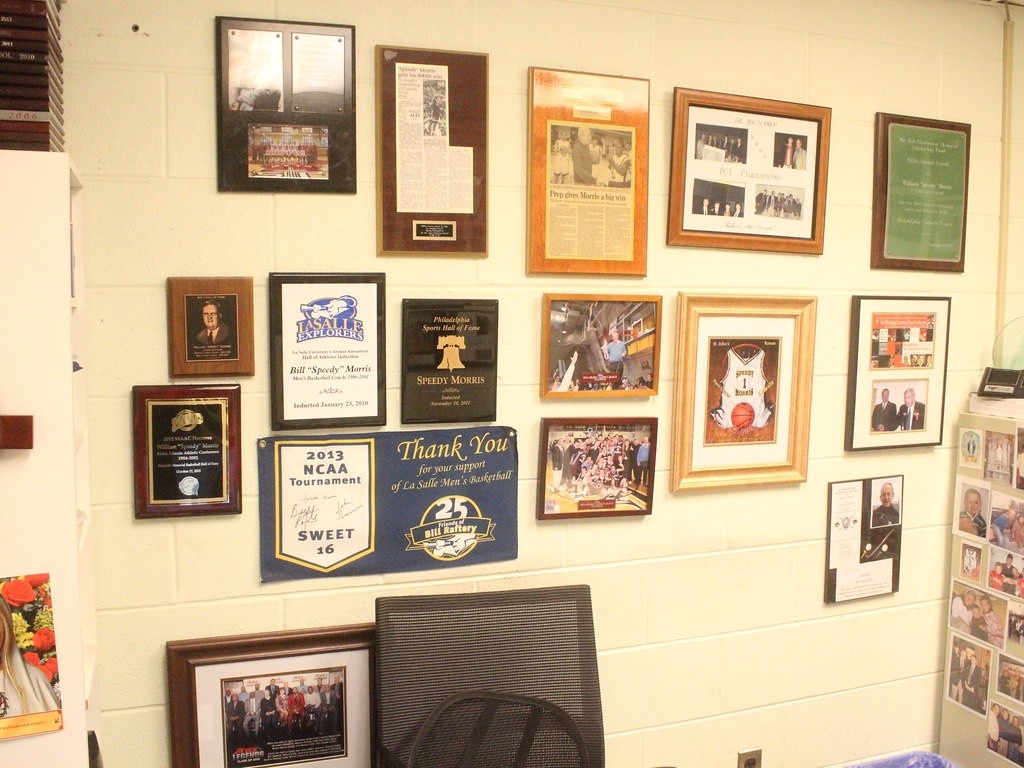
[731,403,755,429]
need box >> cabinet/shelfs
[0,151,94,768]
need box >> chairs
[374,585,605,768]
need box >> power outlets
[738,747,762,768]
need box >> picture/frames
[870,112,971,272]
[167,276,254,377]
[539,293,663,399]
[167,621,376,768]
[524,67,651,276]
[668,291,818,493]
[131,384,245,519]
[844,295,952,452]
[666,86,833,255]
[374,43,490,259]
[270,271,386,430]
[535,417,658,518]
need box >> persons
[225,676,344,744]
[958,488,986,539]
[607,331,627,390]
[993,509,1024,554]
[696,132,746,163]
[697,198,744,218]
[872,482,899,526]
[988,554,1024,600]
[779,137,806,170]
[871,388,898,431]
[554,131,573,184]
[949,591,1024,767]
[0,594,60,719]
[572,127,602,186]
[588,136,632,187]
[250,140,317,168]
[549,429,650,500]
[552,438,565,487]
[755,189,801,218]
[896,388,925,430]
[551,368,652,391]
